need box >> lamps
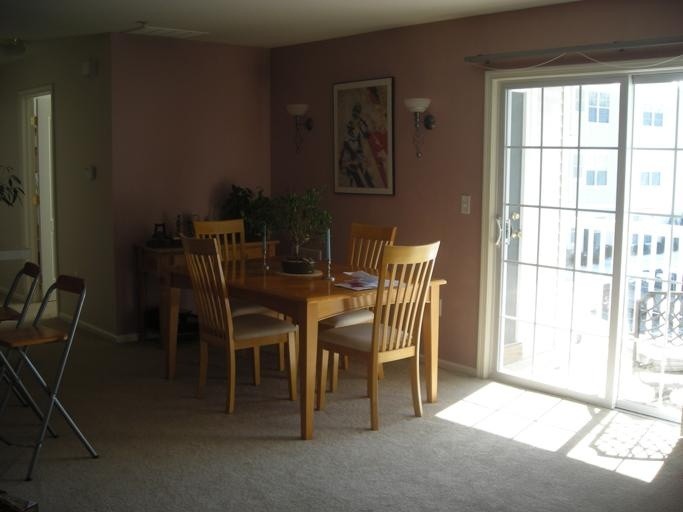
[403,97,435,159]
[287,104,314,153]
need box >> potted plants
[256,184,333,274]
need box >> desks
[166,253,448,440]
[130,235,280,378]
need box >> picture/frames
[330,76,394,196]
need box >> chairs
[0,274,99,482]
[0,262,43,408]
[318,240,441,430]
[318,222,396,391]
[179,238,297,415]
[193,218,285,372]
